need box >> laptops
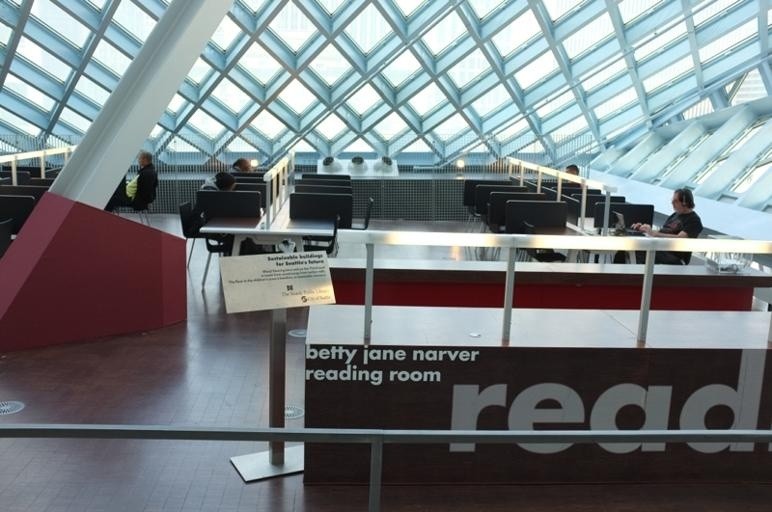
[612,210,643,235]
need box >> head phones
[681,188,689,206]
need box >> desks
[199,174,338,290]
[464,177,669,236]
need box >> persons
[105,150,158,212]
[614,189,703,265]
[565,165,580,177]
[189,158,254,254]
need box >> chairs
[179,197,373,289]
[112,180,159,225]
[512,218,566,262]
